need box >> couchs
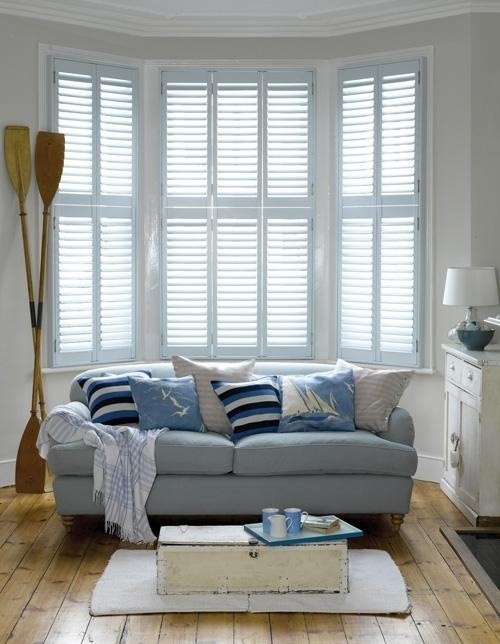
[45,357,418,538]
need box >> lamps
[442,266,498,343]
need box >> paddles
[3,123,50,423]
[14,131,67,494]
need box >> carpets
[245,549,413,615]
[89,546,249,616]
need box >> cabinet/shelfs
[439,343,500,528]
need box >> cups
[268,515,292,538]
[283,508,308,532]
[262,508,280,533]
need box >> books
[301,515,340,534]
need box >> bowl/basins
[456,329,495,351]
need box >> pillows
[334,358,416,433]
[76,370,152,425]
[127,375,208,432]
[277,372,356,430]
[211,375,281,440]
[172,355,255,435]
